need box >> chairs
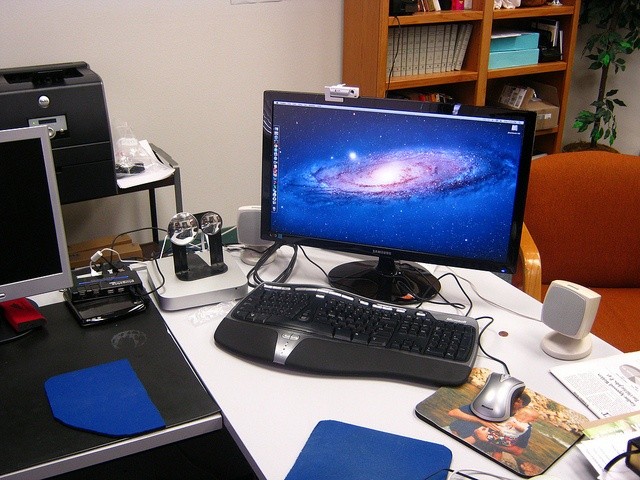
[510,150,640,353]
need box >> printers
[0,62,118,209]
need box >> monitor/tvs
[260,90,537,306]
[0,126,73,344]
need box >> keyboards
[214,279,480,387]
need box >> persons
[464,407,536,459]
[438,390,533,458]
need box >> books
[387,91,453,104]
[415,0,472,11]
[386,24,474,77]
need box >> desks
[1,142,221,478]
[133,242,640,480]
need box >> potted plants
[561,1,640,157]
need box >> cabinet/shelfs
[344,0,581,159]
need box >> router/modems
[147,248,250,313]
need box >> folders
[388,24,472,78]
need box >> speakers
[235,204,273,266]
[540,277,602,362]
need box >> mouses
[469,373,526,423]
